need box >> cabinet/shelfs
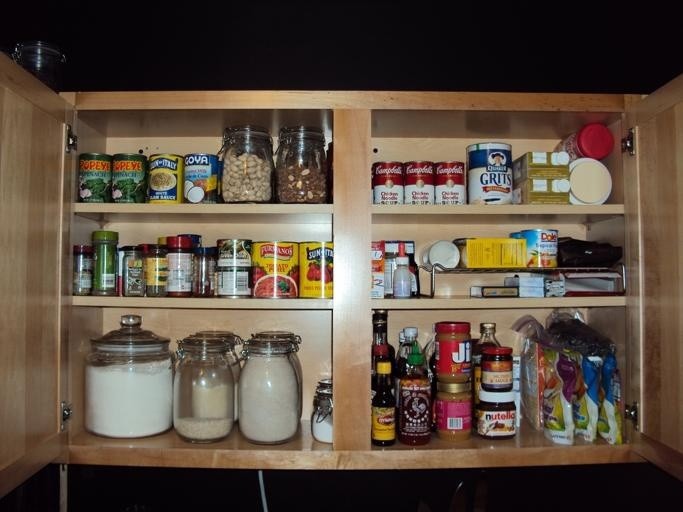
[0,49,683,498]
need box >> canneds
[435,321,517,442]
[73,230,334,299]
[463,141,517,203]
[75,153,219,206]
[509,229,558,269]
[373,159,468,206]
[422,240,460,272]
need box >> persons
[492,153,504,166]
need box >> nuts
[222,147,327,204]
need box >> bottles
[217,125,274,203]
[81,315,332,448]
[72,229,217,299]
[273,126,330,203]
[471,323,501,428]
[392,240,411,298]
[372,308,432,448]
